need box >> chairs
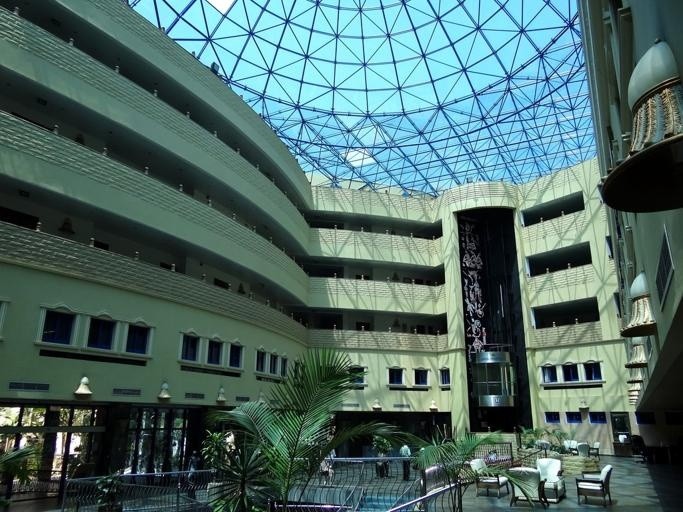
[534,438,600,460]
[469,457,562,509]
[574,464,613,508]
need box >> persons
[399,442,412,481]
[186,450,201,482]
[316,454,330,485]
[329,447,337,469]
